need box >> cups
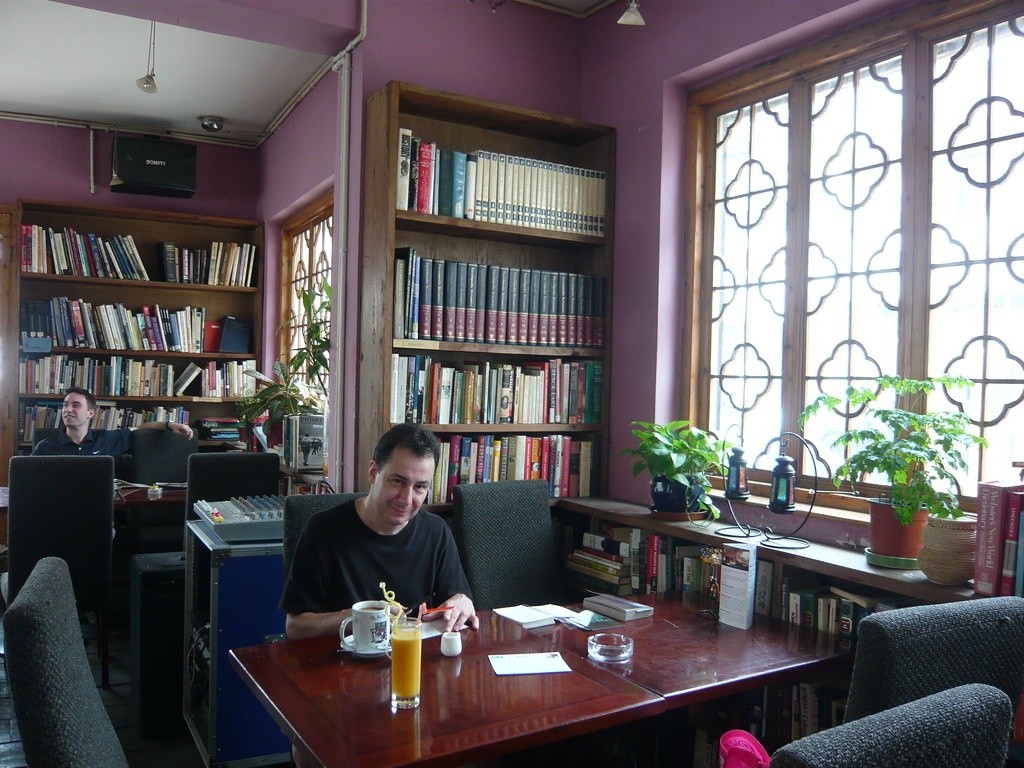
[392,618,423,709]
[340,601,388,650]
[440,632,462,656]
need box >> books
[974,462,1024,745]
[19,225,256,445]
[494,513,939,768]
[391,128,605,503]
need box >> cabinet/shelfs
[557,497,1024,768]
[184,519,292,768]
[9,197,265,455]
[356,81,618,519]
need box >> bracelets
[166,422,173,432]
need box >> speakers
[110,137,197,198]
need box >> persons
[277,422,480,642]
[32,387,193,621]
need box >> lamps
[136,21,158,94]
[715,424,761,539]
[752,433,818,549]
[109,129,124,186]
[616,0,645,26]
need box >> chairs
[842,595,1024,727]
[125,429,199,557]
[283,490,371,579]
[0,453,114,688]
[2,557,129,768]
[450,480,573,611]
[769,683,1013,768]
[181,453,282,558]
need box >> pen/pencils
[404,592,436,613]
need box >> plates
[340,634,391,658]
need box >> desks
[494,589,857,768]
[0,487,124,513]
[228,611,667,768]
[115,485,187,507]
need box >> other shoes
[86,611,96,623]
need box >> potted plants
[619,420,735,527]
[795,374,989,571]
